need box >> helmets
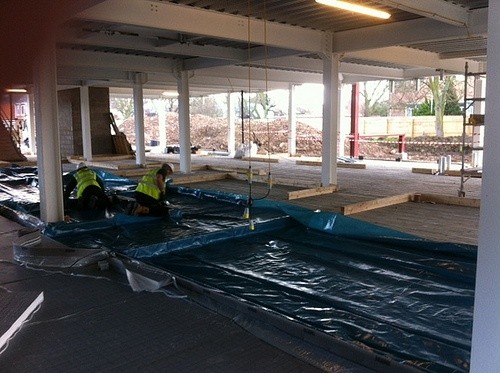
[76,162,88,171]
[165,162,175,173]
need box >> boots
[130,203,149,217]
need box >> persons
[63,162,112,217]
[136,162,176,201]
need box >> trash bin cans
[153,141,157,145]
[149,140,153,146]
